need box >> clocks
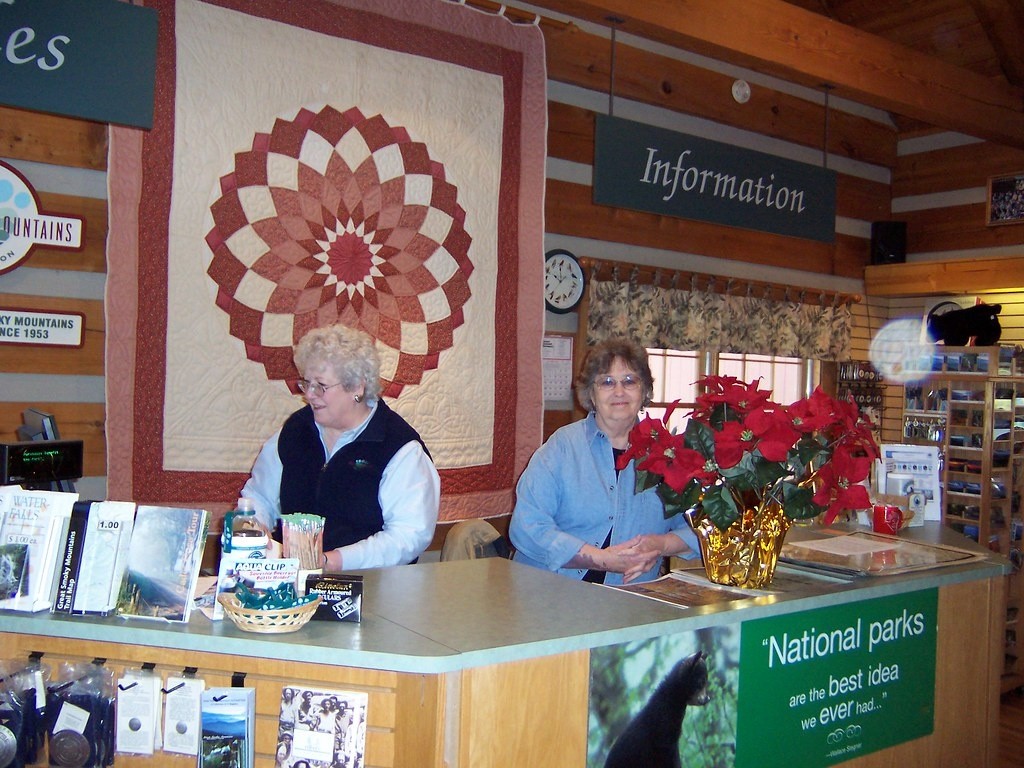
[545,247,587,314]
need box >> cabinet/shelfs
[902,340,1024,696]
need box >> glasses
[296,378,341,398]
[591,375,644,392]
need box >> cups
[873,503,904,536]
[298,569,323,596]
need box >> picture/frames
[985,170,1024,226]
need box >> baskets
[867,505,915,532]
[217,592,323,634]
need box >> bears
[602,650,713,768]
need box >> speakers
[871,221,906,265]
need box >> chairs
[440,519,511,561]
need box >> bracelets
[323,553,329,564]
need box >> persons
[508,337,702,586]
[275,687,365,768]
[232,324,441,572]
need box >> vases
[681,497,793,590]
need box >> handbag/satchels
[927,301,1002,346]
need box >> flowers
[616,374,881,527]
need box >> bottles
[216,496,270,612]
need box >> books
[0,485,211,624]
